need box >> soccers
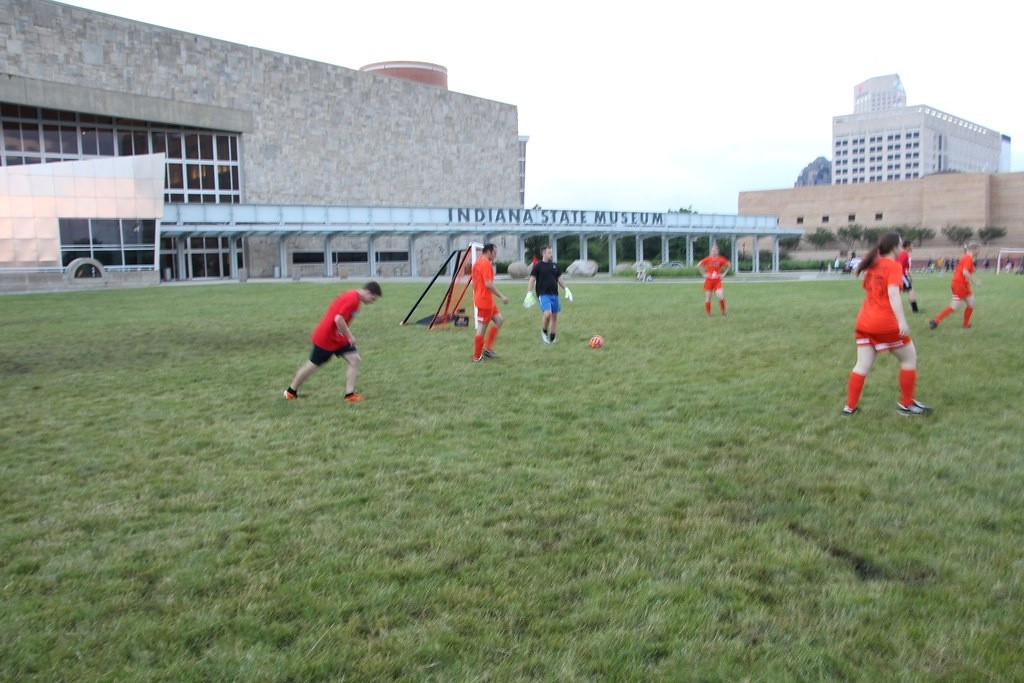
[589,334,605,350]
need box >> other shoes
[929,319,937,331]
[541,330,550,345]
[551,337,558,343]
[472,353,483,362]
[283,390,299,400]
[344,394,363,401]
[483,348,497,358]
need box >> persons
[842,232,935,419]
[928,242,981,330]
[472,243,509,362]
[697,246,731,319]
[895,240,919,313]
[522,244,573,344]
[282,281,382,402]
[820,249,1024,276]
[636,263,647,282]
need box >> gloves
[564,288,574,302]
[523,290,537,309]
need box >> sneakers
[842,405,860,416]
[896,399,933,417]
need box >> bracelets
[703,274,707,278]
